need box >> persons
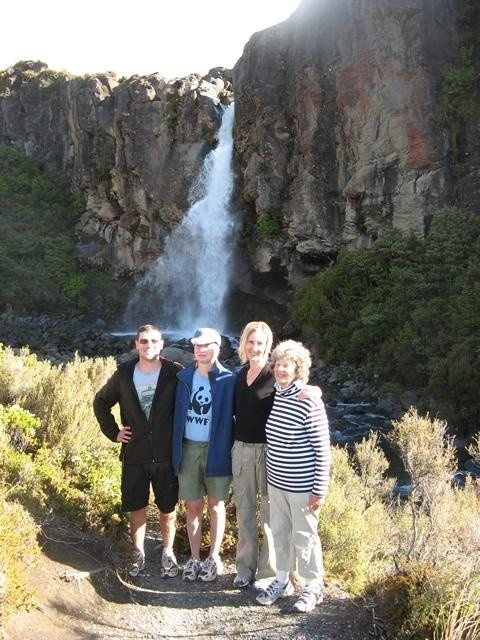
[92,322,185,581]
[253,337,333,615]
[173,326,238,583]
[226,319,278,594]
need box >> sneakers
[129,552,146,576]
[160,554,178,578]
[198,558,217,582]
[256,580,294,605]
[182,558,201,582]
[252,576,274,591]
[292,585,323,612]
[233,574,255,588]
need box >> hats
[190,328,221,346]
